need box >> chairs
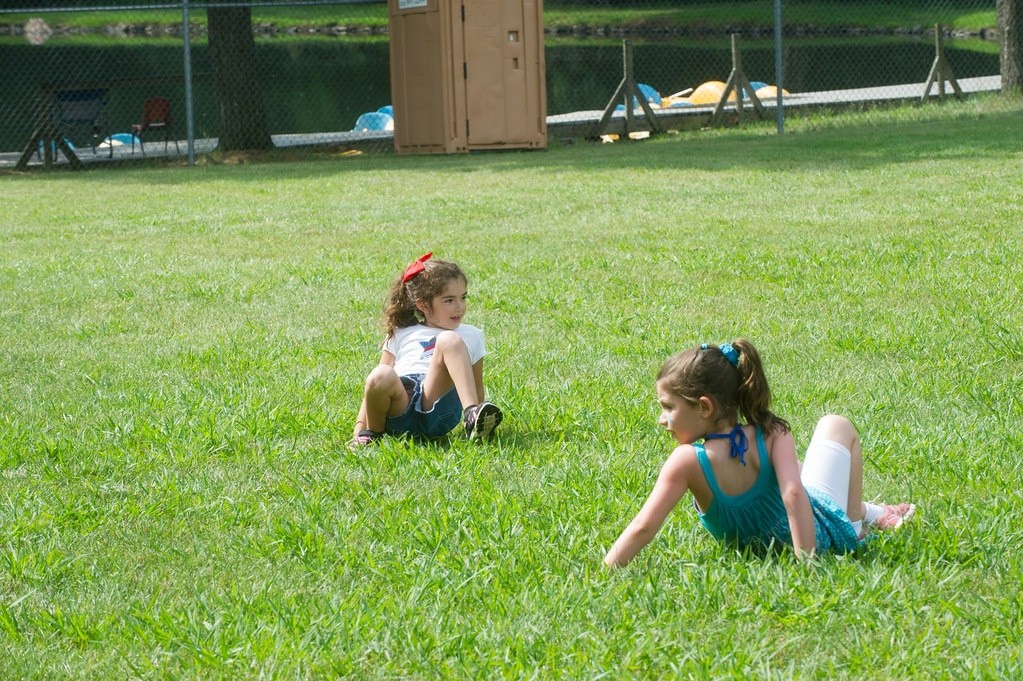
[130,96,181,158]
[36,78,115,160]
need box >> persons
[595,338,918,574]
[352,252,504,446]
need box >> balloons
[354,105,395,131]
[98,133,142,149]
[615,81,791,111]
[38,136,75,154]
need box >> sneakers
[348,430,379,454]
[858,501,917,542]
[463,401,503,445]
